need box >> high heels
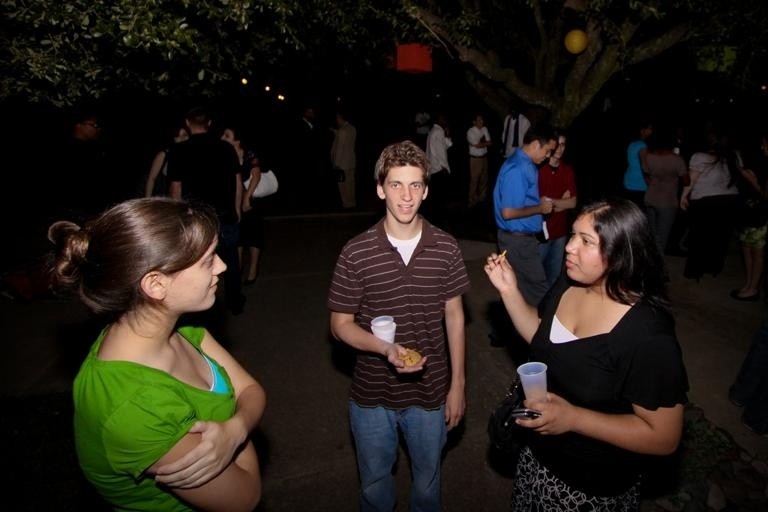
[243,265,261,286]
[730,290,759,302]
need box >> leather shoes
[233,292,246,318]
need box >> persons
[642,133,689,259]
[46,199,268,509]
[425,111,453,213]
[704,117,766,302]
[244,215,250,217]
[622,121,654,205]
[482,196,686,511]
[328,108,361,212]
[492,122,553,310]
[680,127,738,282]
[465,112,491,208]
[168,107,250,317]
[221,127,262,212]
[329,140,465,512]
[535,127,577,285]
[500,98,532,158]
[144,126,191,201]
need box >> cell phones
[512,408,540,420]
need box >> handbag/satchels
[488,356,534,474]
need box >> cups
[371,315,396,344]
[517,362,548,404]
